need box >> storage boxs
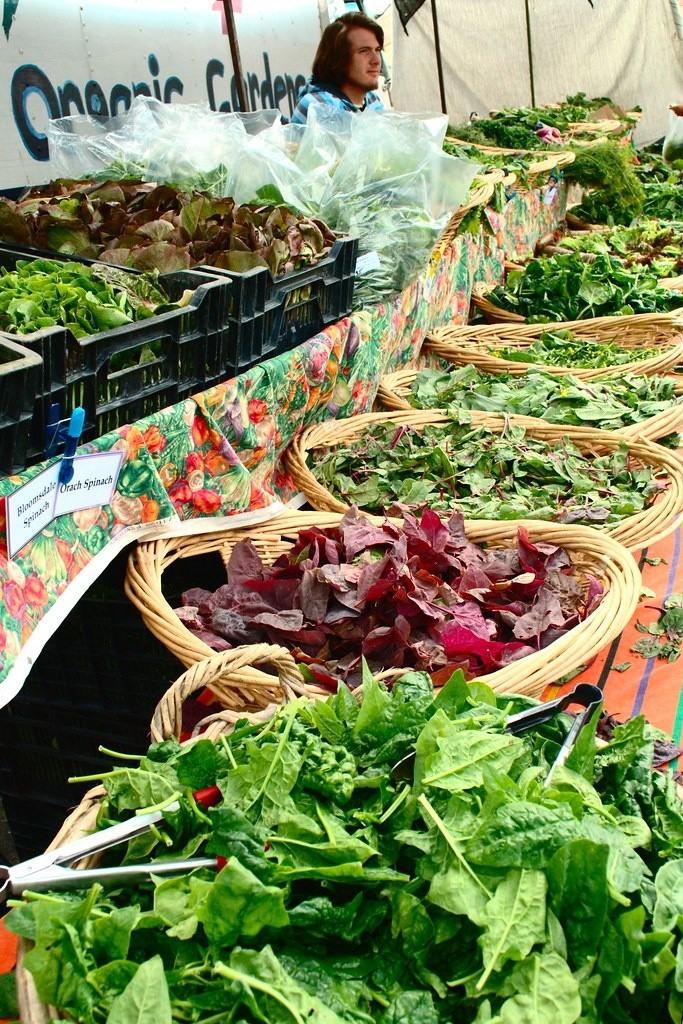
[0,179,359,478]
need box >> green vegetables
[1,93,683,1024]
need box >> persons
[287,11,390,147]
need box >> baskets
[15,639,304,1024]
[0,179,360,478]
[442,99,642,195]
[430,177,492,265]
[128,516,643,713]
[281,407,683,556]
[382,229,683,446]
